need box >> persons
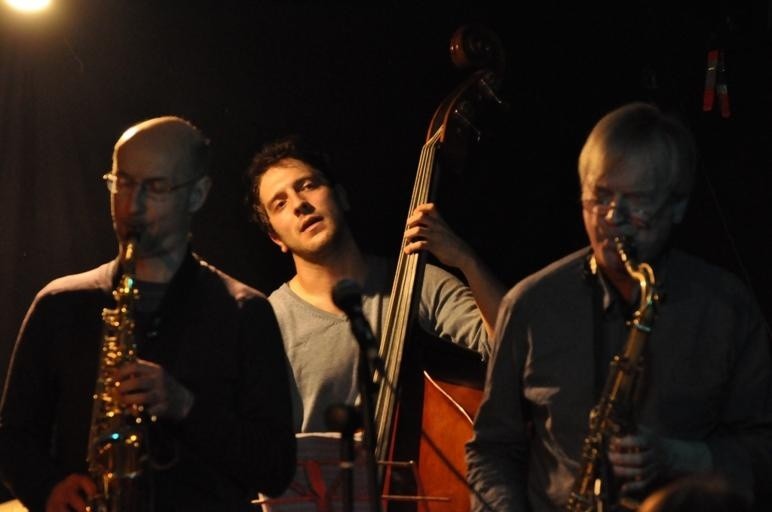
[463,101,772,512]
[244,140,509,512]
[0,116,304,512]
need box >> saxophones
[81,234,157,511]
[564,234,754,512]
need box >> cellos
[257,31,500,512]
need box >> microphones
[331,277,387,379]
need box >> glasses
[103,170,193,202]
